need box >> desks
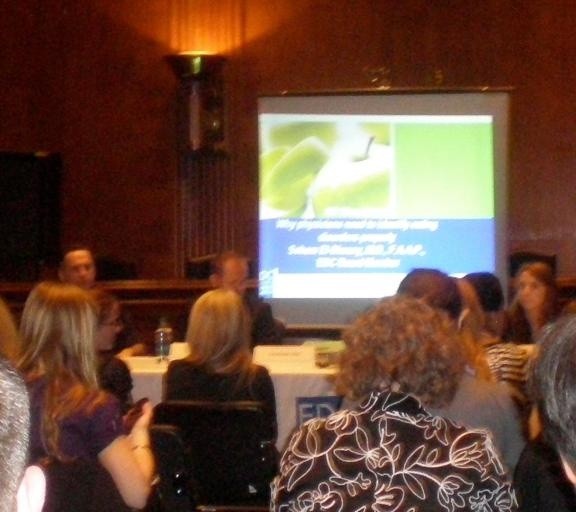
[110,340,341,450]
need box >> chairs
[146,399,270,511]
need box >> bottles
[155,316,173,363]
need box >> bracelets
[127,444,154,451]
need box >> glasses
[102,317,121,326]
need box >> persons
[266,288,525,512]
[52,238,139,355]
[15,276,157,512]
[147,286,282,511]
[0,296,36,511]
[457,270,505,348]
[505,261,575,346]
[526,300,576,491]
[392,266,527,484]
[85,285,131,416]
[175,246,282,346]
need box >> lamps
[170,53,222,164]
